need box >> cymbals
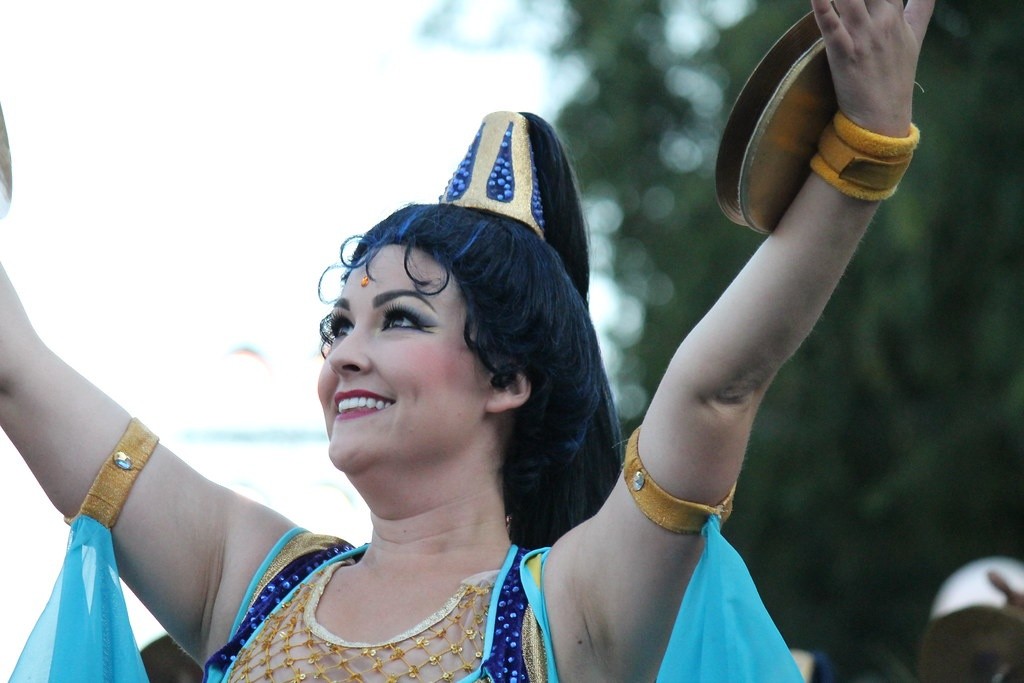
[712,12,838,231]
[0,104,11,222]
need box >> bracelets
[812,118,918,199]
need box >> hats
[930,555,1024,624]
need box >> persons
[1,0,942,682]
